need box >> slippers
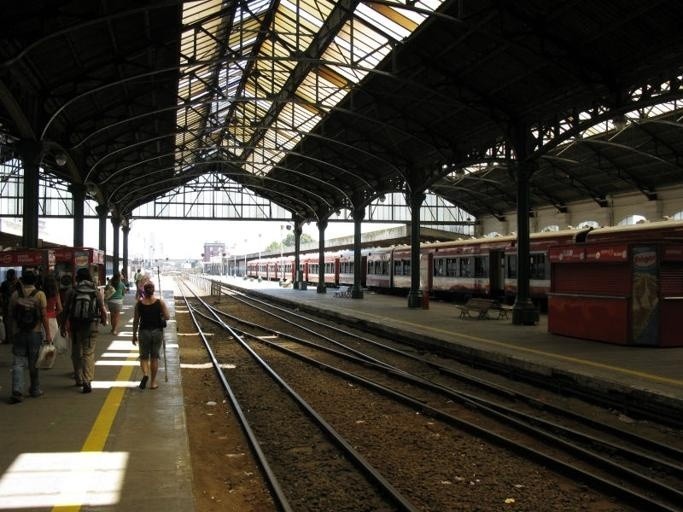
[150,385,159,389]
[139,375,149,389]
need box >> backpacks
[14,287,41,329]
[70,280,98,323]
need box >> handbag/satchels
[104,285,116,300]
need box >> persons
[130,281,172,391]
[0,266,152,347]
[56,267,112,395]
[7,270,53,405]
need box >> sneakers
[12,391,24,402]
[31,389,44,397]
[75,374,92,393]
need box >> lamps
[54,148,69,170]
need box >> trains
[243,216,682,350]
